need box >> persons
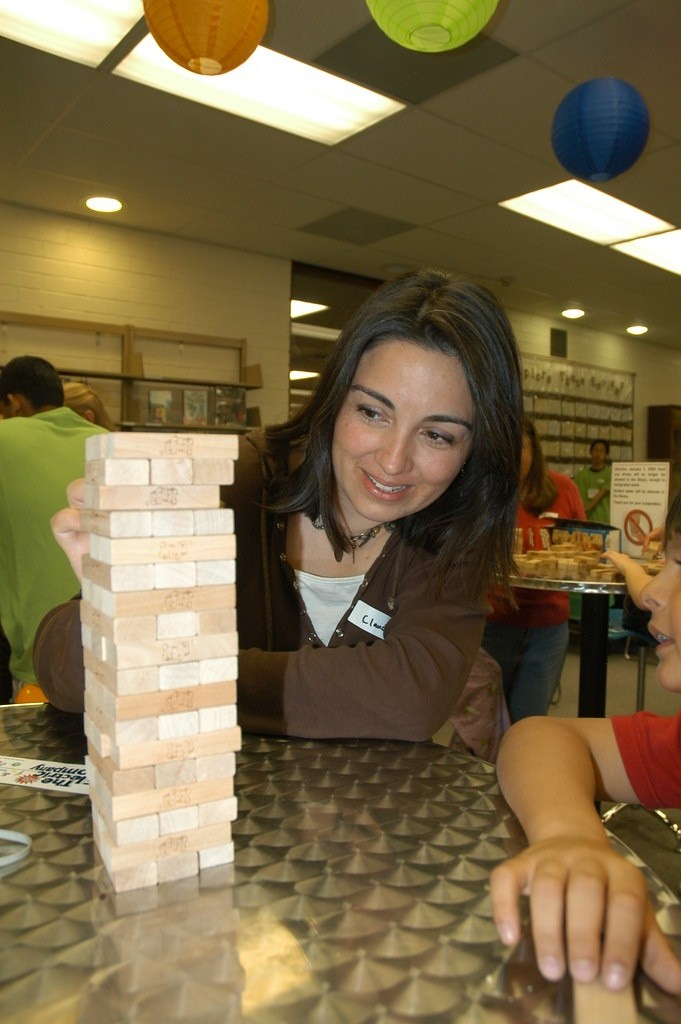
[482,417,586,724]
[491,491,681,999]
[0,355,112,700]
[61,382,122,432]
[574,439,611,526]
[32,271,524,744]
[601,525,665,612]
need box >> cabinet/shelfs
[0,310,265,436]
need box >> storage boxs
[547,517,621,562]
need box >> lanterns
[551,77,649,184]
[143,0,268,75]
[366,0,499,53]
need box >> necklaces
[304,507,380,542]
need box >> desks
[492,573,653,718]
[0,704,681,1024]
